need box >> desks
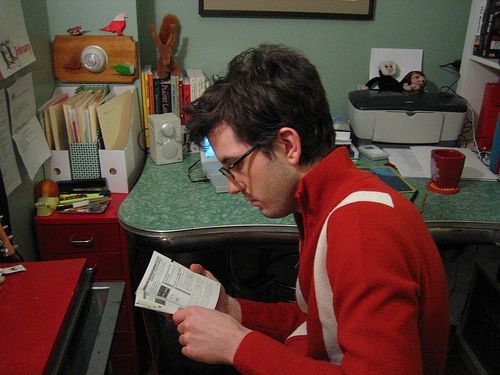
[119,153,500,252]
[0,248,129,375]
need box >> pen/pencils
[56,192,108,211]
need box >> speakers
[147,114,184,164]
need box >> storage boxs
[41,86,144,193]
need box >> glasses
[218,142,261,181]
[188,158,210,183]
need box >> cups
[430,148,466,188]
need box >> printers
[347,90,469,146]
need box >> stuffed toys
[366,60,427,92]
[151,14,181,78]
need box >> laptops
[199,134,231,193]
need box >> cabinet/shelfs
[456,0,500,186]
[34,194,161,375]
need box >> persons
[172,44,450,375]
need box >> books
[141,66,211,148]
[472,0,500,59]
[134,252,222,316]
[383,146,498,182]
